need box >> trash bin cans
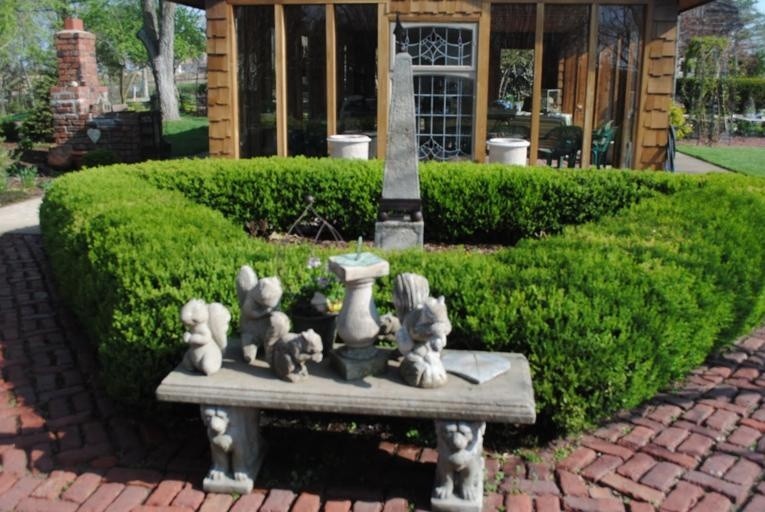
[328,135,372,160]
[487,138,530,164]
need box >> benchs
[154,349,533,511]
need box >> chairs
[526,118,618,169]
